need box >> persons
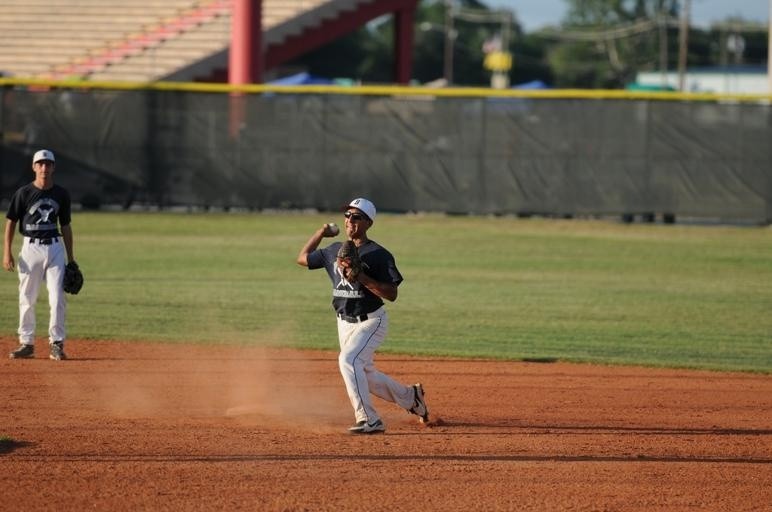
[3,150,73,361]
[296,198,430,434]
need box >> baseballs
[329,224,338,232]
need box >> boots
[50,342,64,361]
[8,344,34,358]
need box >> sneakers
[406,383,429,423]
[347,416,384,432]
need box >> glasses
[344,211,365,220]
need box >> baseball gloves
[336,240,363,284]
[64,260,83,294]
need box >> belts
[337,314,368,323]
[29,237,58,244]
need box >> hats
[33,150,55,162]
[348,198,376,222]
